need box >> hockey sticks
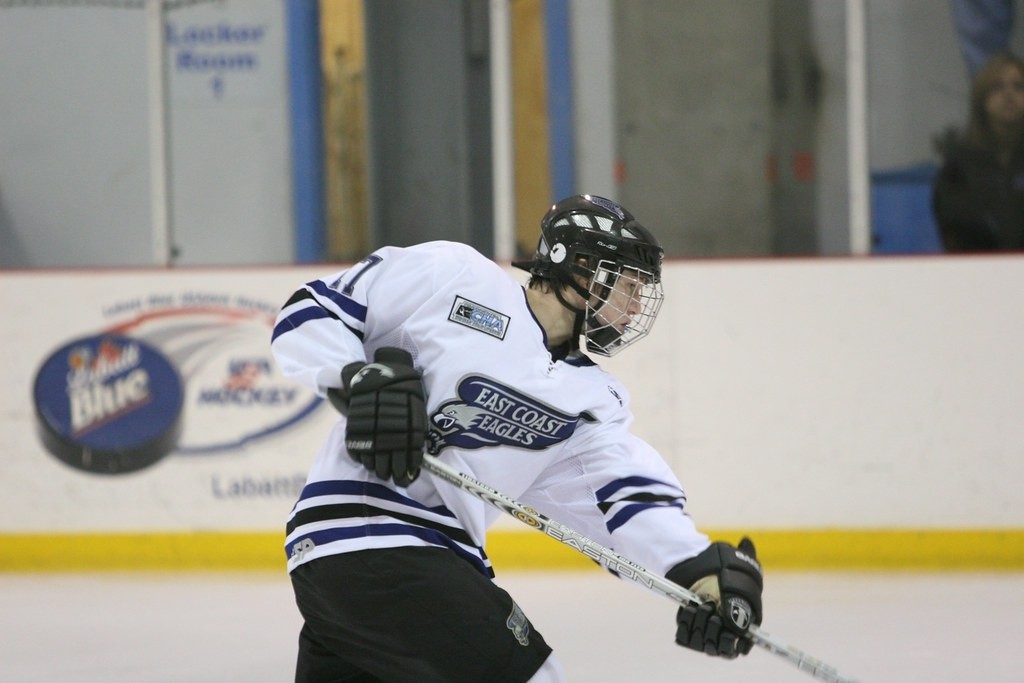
[423,454,856,682]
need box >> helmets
[512,194,664,358]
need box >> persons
[270,193,765,683]
[932,52,1023,250]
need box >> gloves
[330,347,431,488]
[665,535,764,661]
